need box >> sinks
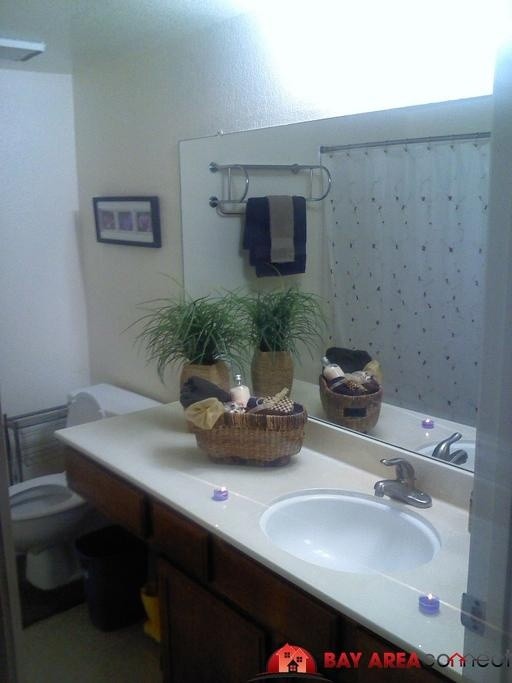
[417,442,476,472]
[264,495,435,576]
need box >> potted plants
[220,282,326,408]
[121,272,255,410]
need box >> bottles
[319,355,345,381]
[229,373,251,406]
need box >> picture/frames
[93,194,160,248]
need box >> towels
[244,194,308,276]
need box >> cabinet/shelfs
[59,448,150,541]
[208,159,334,213]
[151,505,429,682]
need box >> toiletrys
[230,374,251,408]
[320,355,345,383]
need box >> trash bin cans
[73,524,154,632]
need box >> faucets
[431,431,469,467]
[371,455,431,510]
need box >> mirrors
[180,93,492,484]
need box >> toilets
[8,381,166,593]
